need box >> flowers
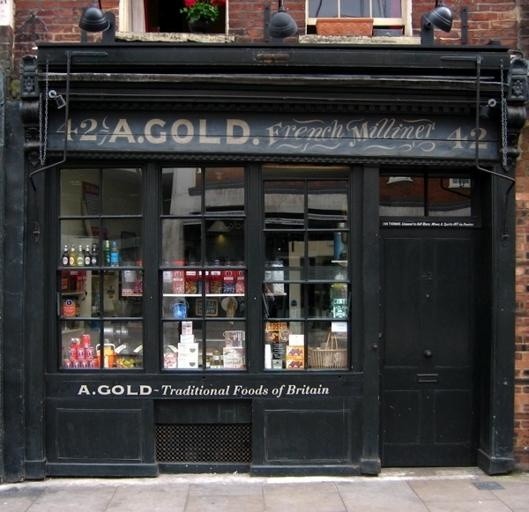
[180,0,225,24]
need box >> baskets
[307,332,347,369]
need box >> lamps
[208,221,231,233]
[81,0,111,33]
[268,0,298,38]
[423,7,455,32]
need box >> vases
[187,20,210,33]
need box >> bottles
[335,222,345,259]
[185,257,247,293]
[61,241,120,267]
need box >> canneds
[63,334,96,369]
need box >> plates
[330,260,348,267]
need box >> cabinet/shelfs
[58,261,287,370]
